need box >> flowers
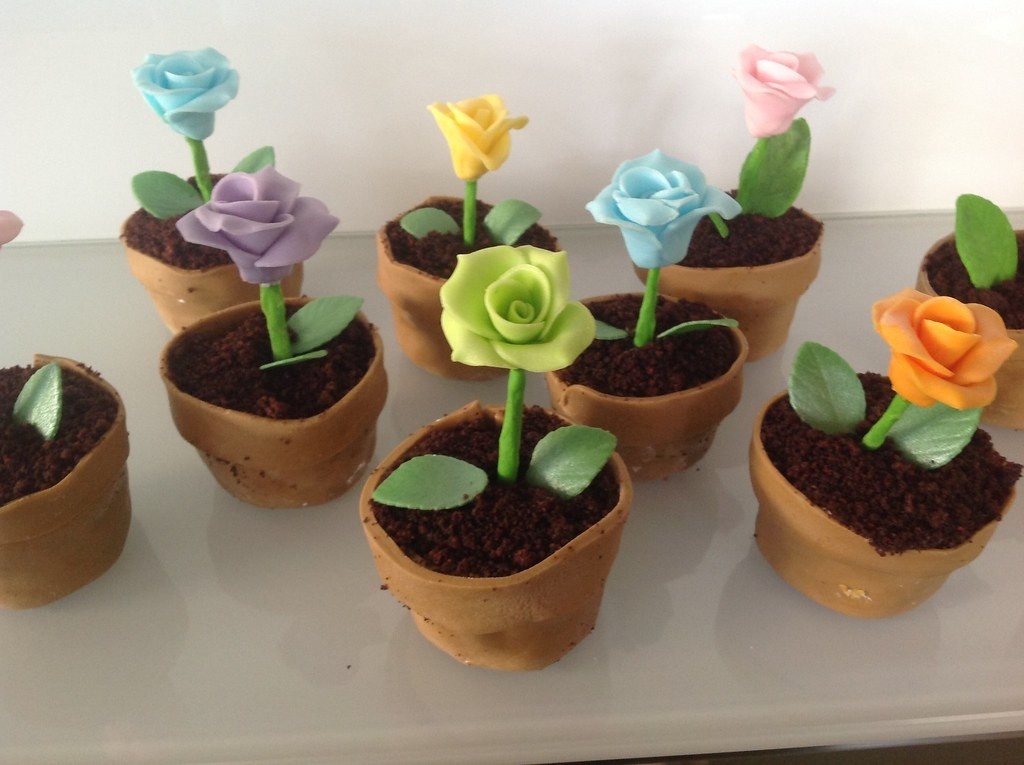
[734,42,834,216]
[177,165,365,368]
[789,286,1017,468]
[400,92,542,247]
[130,46,275,220]
[373,246,618,511]
[586,151,741,348]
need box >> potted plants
[915,195,1024,434]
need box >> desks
[1,206,1024,765]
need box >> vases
[634,185,824,360]
[157,296,389,505]
[120,173,304,335]
[359,401,632,669]
[0,354,132,612]
[380,197,566,382]
[546,293,749,477]
[752,375,1012,617]
